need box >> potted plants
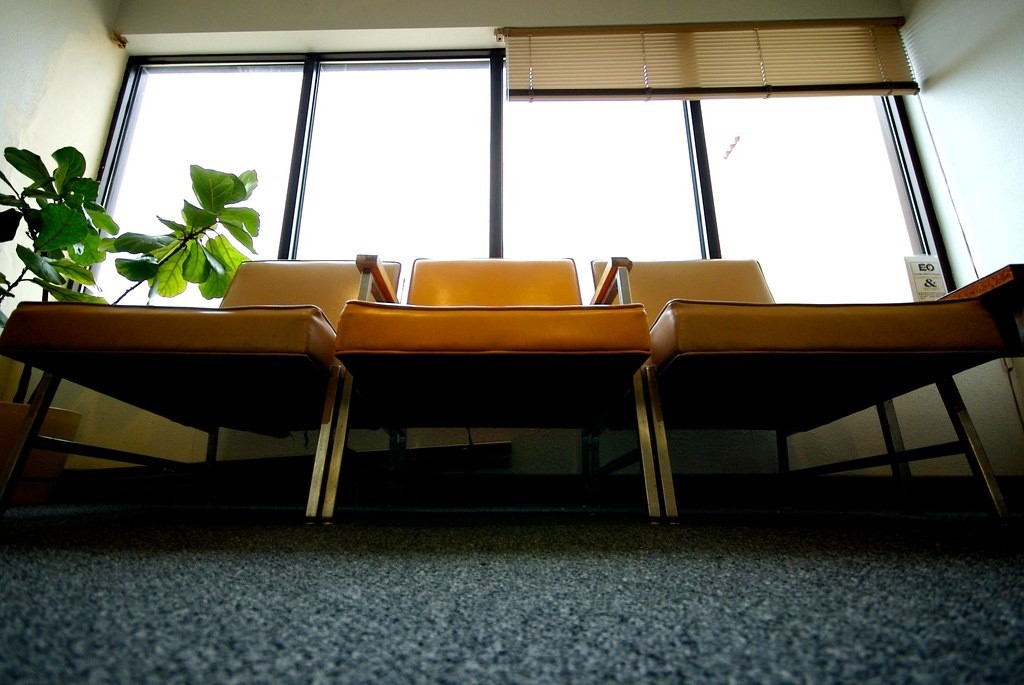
[0,146,261,513]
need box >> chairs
[592,254,1013,533]
[319,256,662,526]
[1,256,401,542]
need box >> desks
[918,265,1024,495]
